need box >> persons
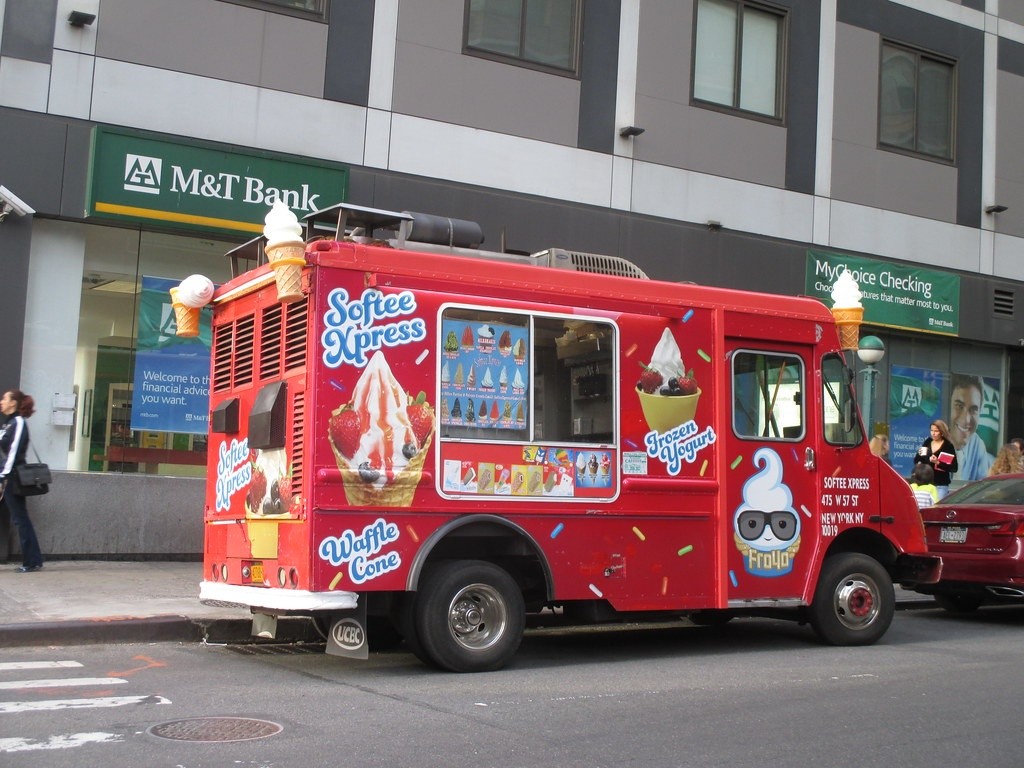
[950,374,989,481]
[1011,438,1024,456]
[869,434,893,467]
[910,465,940,510]
[913,420,958,500]
[0,390,43,573]
[989,443,1024,477]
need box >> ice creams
[496,469,510,490]
[441,361,525,390]
[263,199,306,303]
[576,452,610,486]
[830,270,864,350]
[636,327,702,436]
[440,398,525,432]
[327,350,436,508]
[169,274,214,336]
[245,445,294,559]
[444,325,527,365]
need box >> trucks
[198,203,943,673]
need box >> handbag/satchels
[10,461,53,497]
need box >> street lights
[857,336,885,443]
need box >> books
[935,452,955,472]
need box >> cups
[243,500,292,559]
[635,386,702,435]
[920,447,928,455]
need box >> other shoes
[13,563,42,573]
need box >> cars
[919,473,1024,612]
